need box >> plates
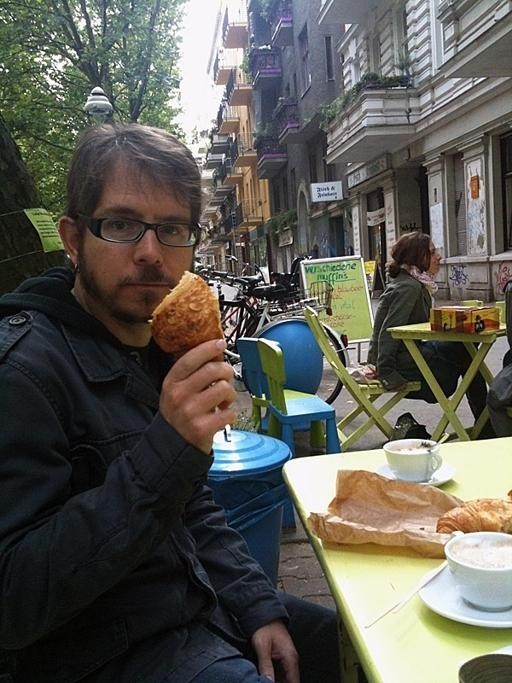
[372,459,455,489]
[418,566,511,629]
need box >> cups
[441,525,511,615]
[382,437,441,483]
[457,653,511,682]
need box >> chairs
[239,304,424,458]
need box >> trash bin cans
[196,425,292,611]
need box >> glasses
[75,212,202,247]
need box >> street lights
[84,83,112,127]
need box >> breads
[436,498,512,533]
[149,268,226,363]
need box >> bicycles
[196,253,350,417]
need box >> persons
[0,124,368,683]
[368,231,488,442]
[487,280,512,439]
